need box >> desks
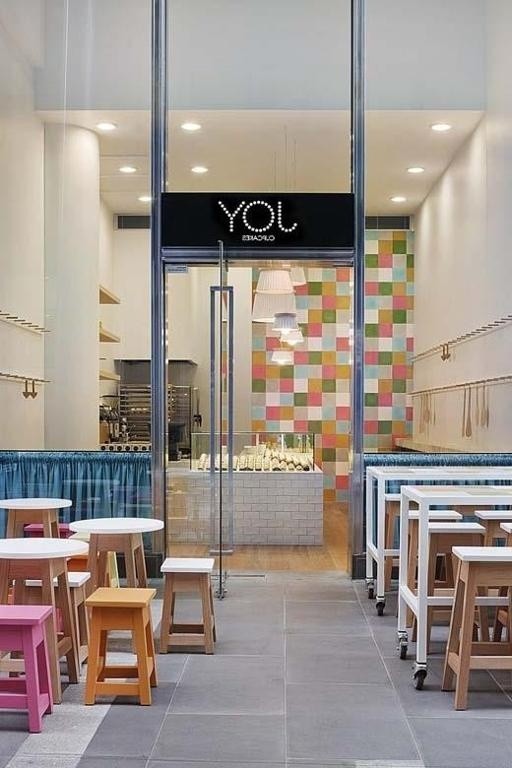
[0,497,72,538]
[366,466,512,616]
[70,518,165,588]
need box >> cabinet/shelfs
[100,285,124,381]
[122,383,192,455]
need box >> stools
[382,492,511,710]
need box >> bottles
[199,443,310,472]
[100,405,128,442]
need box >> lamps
[251,263,304,345]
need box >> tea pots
[192,414,202,433]
